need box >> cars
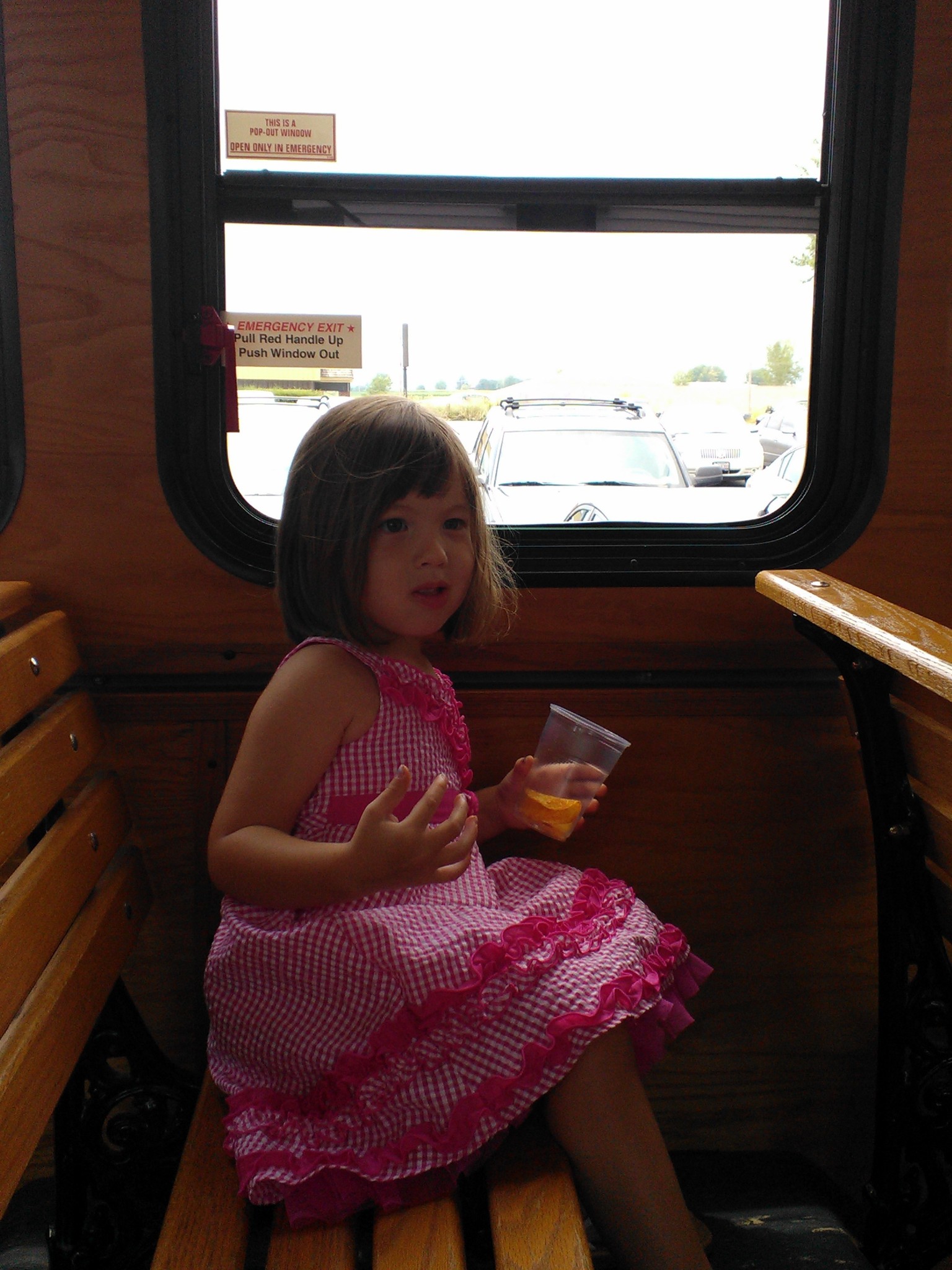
[224,388,356,522]
[755,401,809,464]
[745,442,807,492]
[564,488,793,526]
[655,403,763,485]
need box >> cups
[516,703,633,842]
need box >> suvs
[468,395,695,526]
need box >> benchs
[0,575,589,1269]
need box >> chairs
[752,563,950,1267]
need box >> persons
[197,393,721,1267]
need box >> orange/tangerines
[525,789,581,827]
